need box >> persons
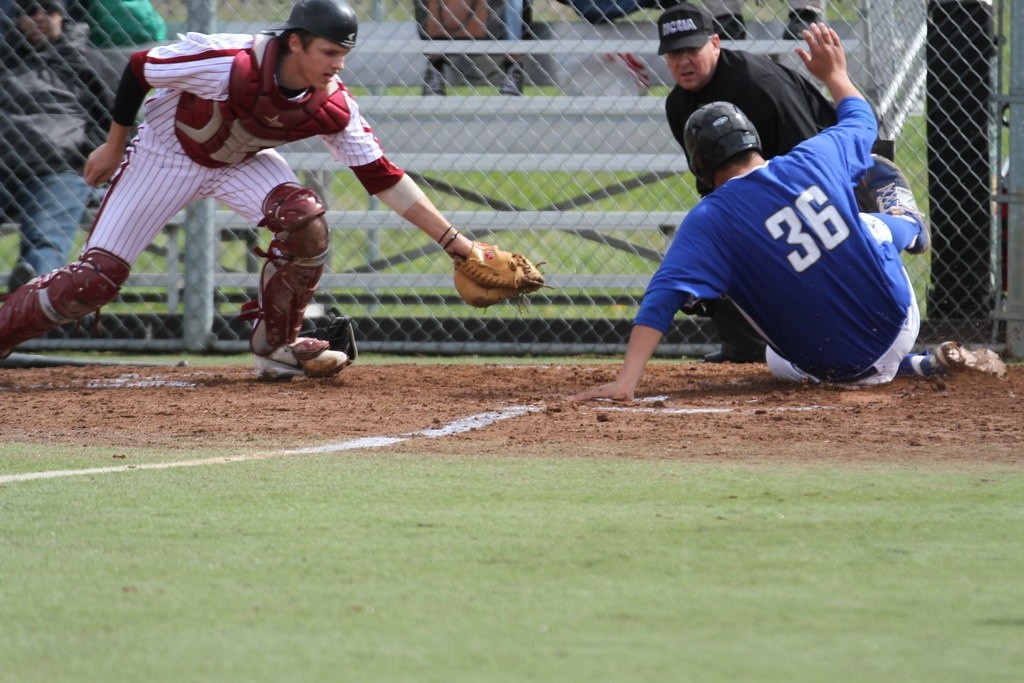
[656,1,841,364]
[0,0,544,380]
[0,0,132,293]
[423,0,532,96]
[707,1,826,40]
[565,20,1008,405]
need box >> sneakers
[863,153,930,255]
[921,341,1009,382]
[251,319,349,377]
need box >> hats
[658,3,714,56]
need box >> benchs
[121,19,873,311]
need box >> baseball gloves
[252,308,357,376]
[453,243,545,307]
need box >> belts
[821,366,879,383]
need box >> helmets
[684,101,761,189]
[269,0,358,48]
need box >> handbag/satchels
[426,0,488,40]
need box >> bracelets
[438,225,460,250]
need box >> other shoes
[500,70,523,96]
[9,263,37,295]
[705,349,763,364]
[423,69,446,96]
[712,15,746,40]
[783,9,822,40]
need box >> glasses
[23,4,57,16]
